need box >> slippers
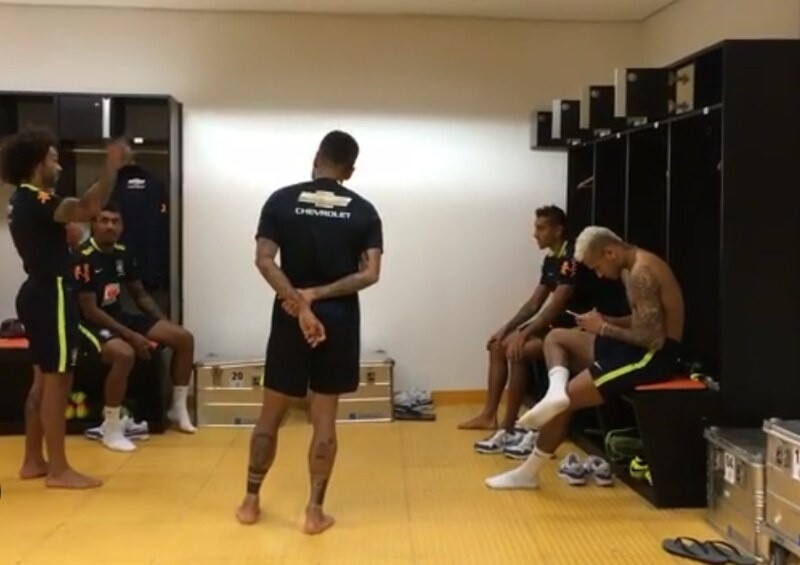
[393,404,435,420]
[662,536,758,564]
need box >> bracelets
[599,322,608,337]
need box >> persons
[72,202,197,453]
[483,226,687,490]
[235,130,384,536]
[456,204,617,452]
[0,125,133,491]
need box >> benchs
[1,334,173,435]
[518,358,708,510]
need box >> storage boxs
[308,348,396,426]
[701,425,769,559]
[195,353,267,428]
[762,416,800,557]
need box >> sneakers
[558,450,586,484]
[473,429,539,458]
[85,415,150,442]
[583,454,615,485]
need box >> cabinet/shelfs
[530,38,794,429]
[2,91,201,335]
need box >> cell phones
[563,309,586,317]
